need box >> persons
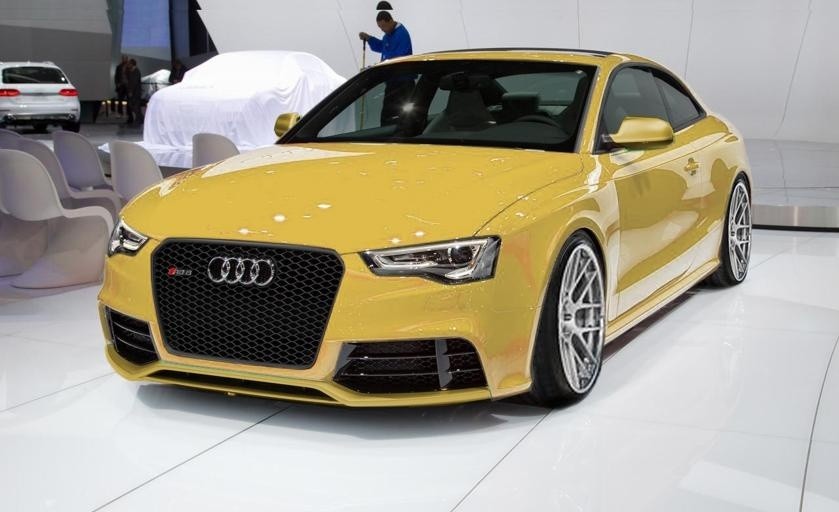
[126,58,144,126]
[109,100,116,114]
[359,12,419,126]
[115,54,130,119]
[168,58,187,84]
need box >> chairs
[103,134,239,200]
[556,76,627,133]
[0,125,120,291]
[422,85,554,134]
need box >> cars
[98,48,751,408]
[0,61,81,132]
